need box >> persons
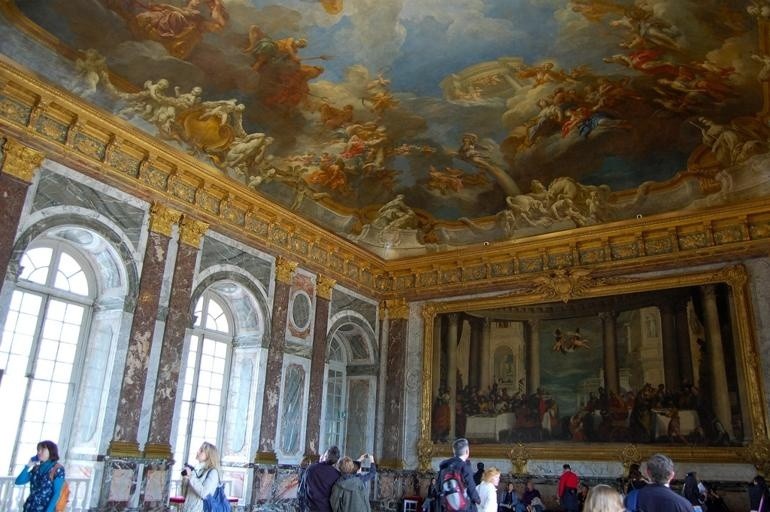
[433,377,730,445]
[421,478,436,512]
[682,472,708,506]
[16,440,69,511]
[298,441,377,511]
[435,437,481,511]
[749,475,770,512]
[552,325,566,352]
[624,453,695,511]
[473,462,500,512]
[552,264,571,298]
[497,480,543,512]
[180,441,219,511]
[499,354,514,381]
[569,329,592,352]
[584,483,626,512]
[558,463,589,512]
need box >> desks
[462,404,705,445]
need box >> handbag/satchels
[55,480,71,512]
[204,483,231,512]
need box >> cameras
[181,463,194,476]
[686,472,695,476]
[364,453,370,458]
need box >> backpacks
[440,461,470,512]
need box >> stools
[401,495,423,511]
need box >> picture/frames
[415,264,770,466]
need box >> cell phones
[31,454,40,461]
